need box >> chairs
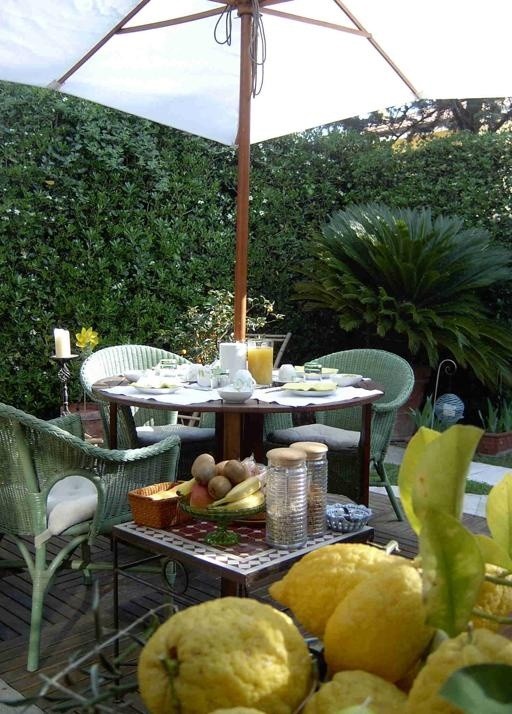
[79,343,216,478]
[0,402,181,678]
[263,348,415,523]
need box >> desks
[113,489,376,707]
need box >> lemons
[409,622,512,713]
[137,597,312,712]
[305,670,409,714]
[471,563,511,635]
[323,560,436,683]
[269,543,415,637]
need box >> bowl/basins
[122,370,142,383]
[328,503,373,532]
[277,363,298,380]
[216,386,254,403]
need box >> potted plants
[477,400,507,455]
[288,203,512,443]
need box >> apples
[190,483,214,509]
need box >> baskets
[127,481,194,529]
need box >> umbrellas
[0,0,512,458]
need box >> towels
[97,384,224,406]
[247,384,383,410]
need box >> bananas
[176,478,197,497]
[207,476,265,510]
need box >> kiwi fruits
[191,454,216,487]
[207,476,232,500]
[223,460,246,485]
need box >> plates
[132,384,186,395]
[179,500,264,546]
[327,374,363,386]
[287,388,337,397]
[269,377,301,386]
[294,366,338,380]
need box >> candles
[53,326,72,359]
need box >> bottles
[290,441,328,539]
[266,448,308,550]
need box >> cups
[248,340,274,388]
[304,362,323,382]
[186,364,203,382]
[219,343,246,374]
[160,359,178,378]
[233,368,257,387]
